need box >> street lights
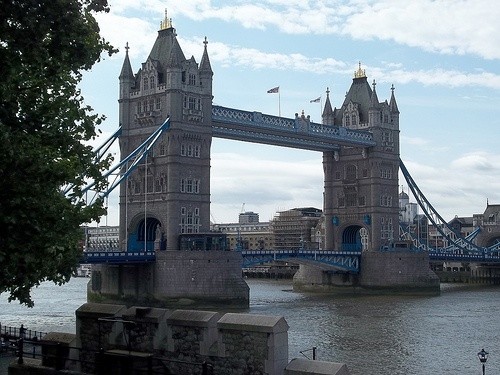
[477,348,490,375]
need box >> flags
[310,97,321,102]
[267,86,278,93]
